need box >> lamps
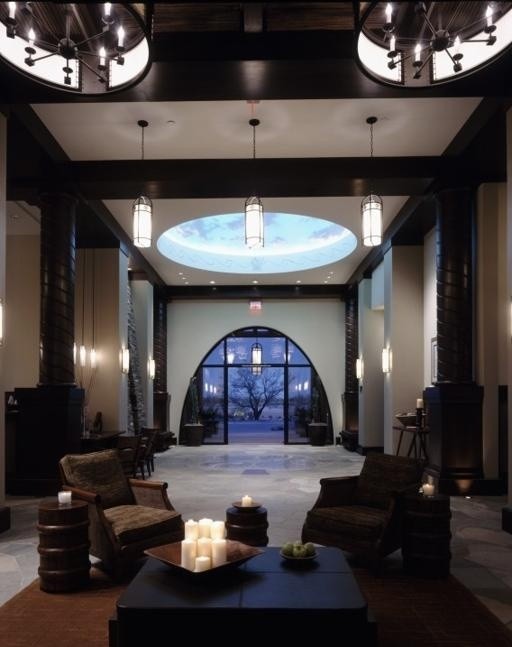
[250,337,262,374]
[132,120,152,247]
[360,117,383,248]
[244,118,265,250]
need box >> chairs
[116,426,159,480]
[58,450,180,578]
[300,453,423,565]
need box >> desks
[83,427,125,441]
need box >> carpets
[0,568,511,647]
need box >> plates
[279,549,319,561]
[231,501,262,509]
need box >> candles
[58,492,72,504]
[350,0,512,81]
[424,485,434,495]
[242,495,252,506]
[180,518,227,571]
[417,399,424,408]
[2,4,151,95]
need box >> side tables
[37,501,89,595]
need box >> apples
[281,543,315,557]
